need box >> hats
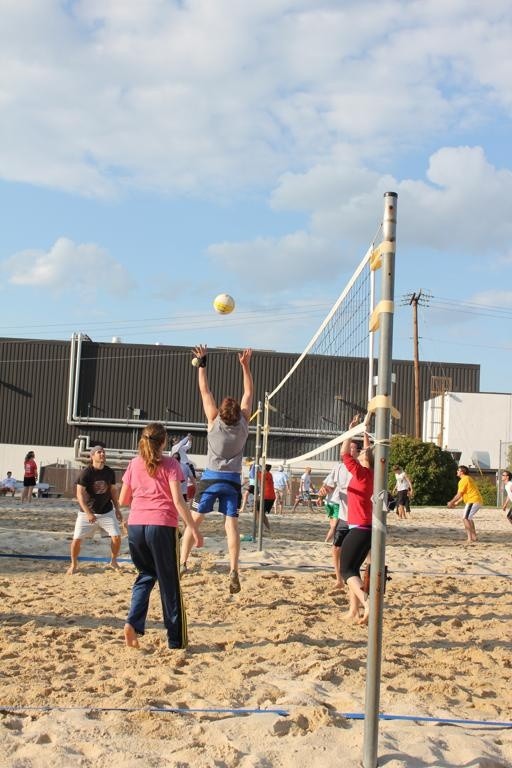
[90,446,103,454]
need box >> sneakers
[231,570,240,593]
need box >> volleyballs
[213,294,235,315]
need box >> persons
[67,446,123,574]
[392,465,413,519]
[116,421,206,648]
[500,469,512,525]
[446,464,484,544]
[239,456,316,530]
[1,471,17,498]
[177,343,255,594]
[318,414,374,623]
[170,432,198,502]
[21,451,38,503]
